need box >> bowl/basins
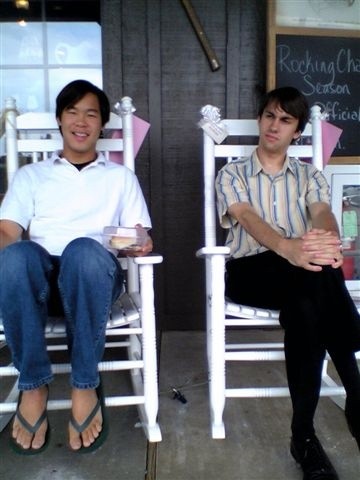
[101,226,149,251]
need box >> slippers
[11,383,51,454]
[69,373,107,453]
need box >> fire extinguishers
[338,193,359,280]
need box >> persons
[0,79,153,455]
[216,86,360,480]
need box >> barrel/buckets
[341,196,356,280]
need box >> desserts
[108,228,136,249]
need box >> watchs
[340,243,343,249]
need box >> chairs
[197,105,359,440]
[0,96,163,443]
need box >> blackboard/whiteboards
[267,26,360,164]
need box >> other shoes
[290,434,339,480]
[345,404,360,452]
